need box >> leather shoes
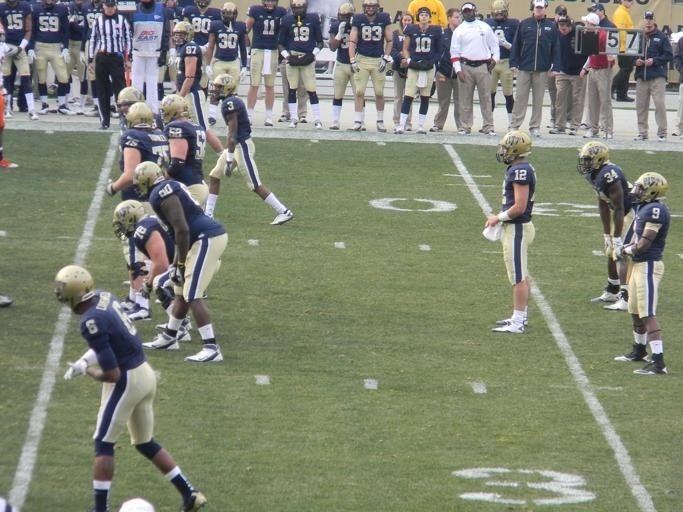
[62,359,87,380]
[27,50,37,64]
[167,54,176,67]
[202,46,207,54]
[60,48,71,63]
[604,234,613,250]
[299,53,315,66]
[206,66,213,78]
[158,50,167,67]
[67,361,85,378]
[612,237,622,247]
[377,58,391,73]
[614,245,631,260]
[170,264,184,286]
[287,55,299,65]
[238,68,246,83]
[139,284,152,299]
[224,160,233,177]
[350,61,360,73]
[7,44,22,57]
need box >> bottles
[103,1,116,7]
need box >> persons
[613,172,670,374]
[576,140,638,311]
[52,264,207,511]
[106,73,294,362]
[1,1,683,143]
[484,131,537,332]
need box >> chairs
[207,118,217,129]
[591,290,621,303]
[4,100,120,121]
[616,96,634,102]
[491,322,525,334]
[269,210,293,225]
[119,298,225,364]
[614,350,650,363]
[0,159,19,168]
[632,360,667,376]
[603,298,628,311]
[180,491,208,512]
[611,80,615,99]
[496,317,528,326]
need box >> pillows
[629,172,668,207]
[114,73,237,131]
[53,265,96,311]
[494,132,532,164]
[133,161,165,198]
[111,199,144,242]
[574,142,610,175]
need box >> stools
[461,59,486,67]
[98,52,121,57]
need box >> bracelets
[280,50,290,58]
[226,152,234,162]
[19,39,29,48]
[582,68,587,71]
[497,210,512,221]
[312,47,320,56]
[624,245,634,254]
